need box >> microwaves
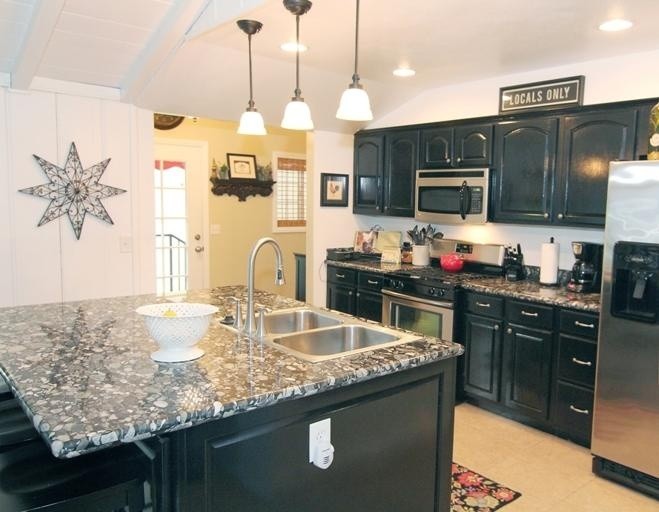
[414,168,488,226]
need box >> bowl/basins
[441,254,464,272]
[135,302,219,363]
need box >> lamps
[336,1,378,120]
[284,1,311,129]
[236,19,267,137]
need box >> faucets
[226,236,286,338]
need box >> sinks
[273,324,402,356]
[243,309,345,334]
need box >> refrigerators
[591,160,659,499]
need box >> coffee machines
[567,242,603,293]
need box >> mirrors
[270,151,309,233]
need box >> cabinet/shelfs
[353,130,420,217]
[492,109,639,225]
[326,267,389,323]
[461,298,553,421]
[549,307,599,448]
[421,124,492,168]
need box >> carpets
[447,461,521,512]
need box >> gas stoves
[383,267,497,301]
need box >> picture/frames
[320,172,350,206]
[227,153,257,181]
